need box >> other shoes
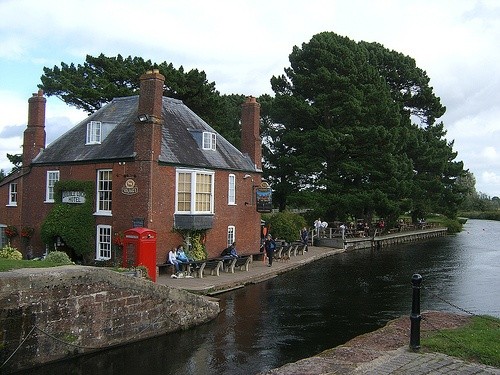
[267,263,272,267]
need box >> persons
[338,217,427,238]
[176,245,200,272]
[302,227,310,252]
[220,242,239,268]
[167,247,184,279]
[314,218,322,238]
[263,234,277,267]
[320,220,329,239]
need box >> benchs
[155,243,307,279]
[338,219,432,239]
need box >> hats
[266,234,272,238]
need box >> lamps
[137,113,149,122]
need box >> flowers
[5,225,34,238]
